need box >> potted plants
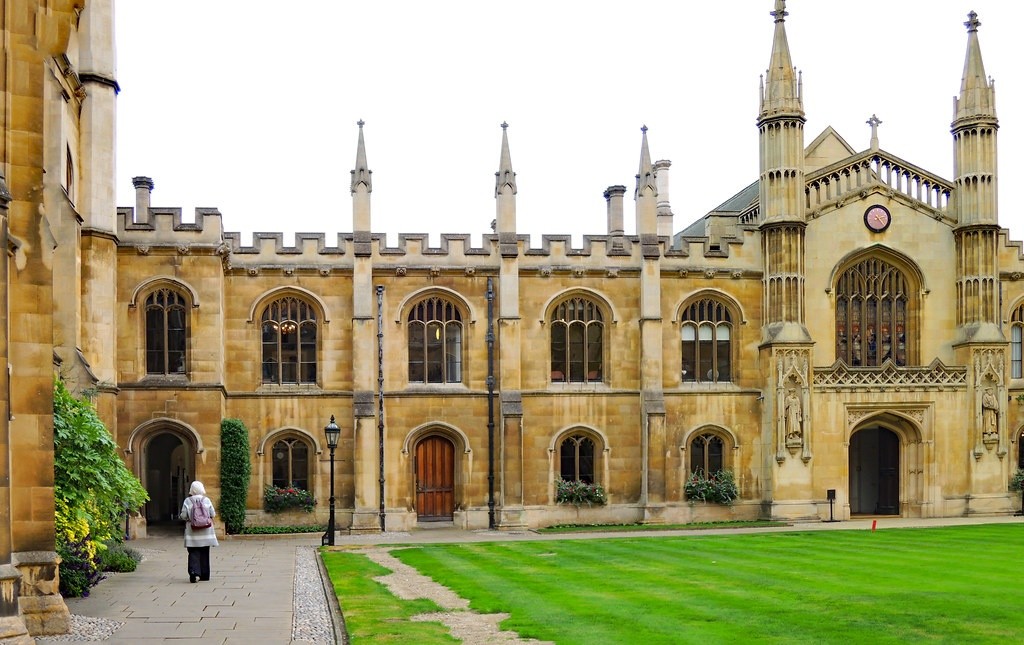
[1010,463,1024,490]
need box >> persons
[180,480,221,583]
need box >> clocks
[864,204,891,233]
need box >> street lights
[320,414,342,543]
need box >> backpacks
[189,496,212,530]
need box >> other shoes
[190,572,197,583]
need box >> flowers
[682,465,739,508]
[264,483,318,514]
[552,473,607,508]
